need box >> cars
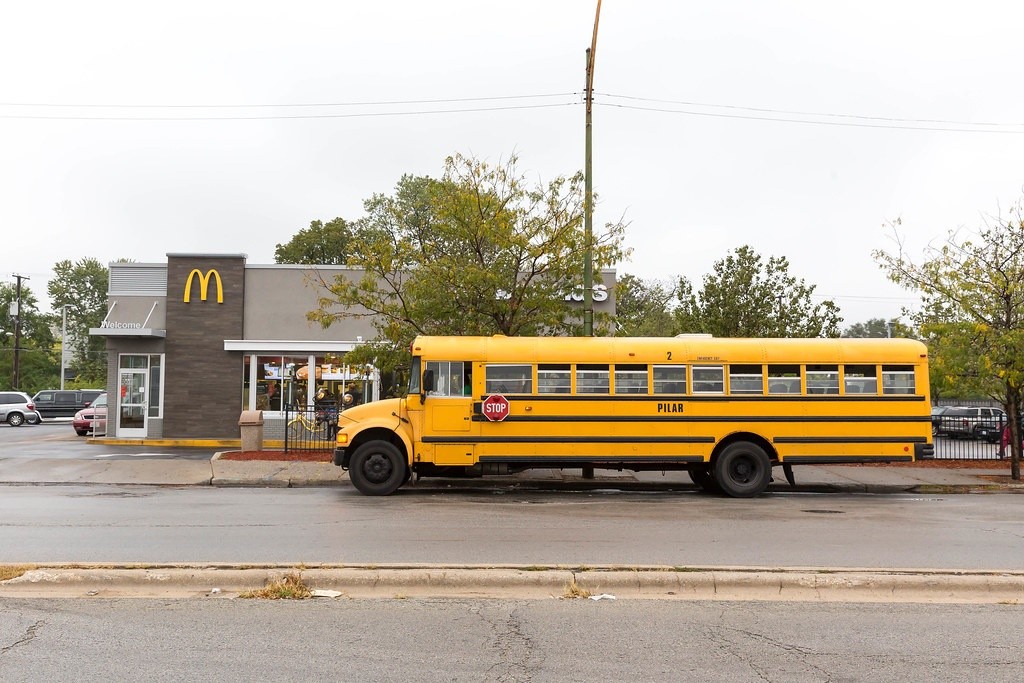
[978,422,1010,443]
[931,405,957,437]
[73,392,137,435]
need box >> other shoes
[996,449,1005,456]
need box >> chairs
[629,383,639,393]
[828,382,840,393]
[639,381,647,393]
[676,383,685,393]
[863,382,877,393]
[715,383,723,391]
[790,381,801,393]
[664,384,676,393]
[555,381,569,394]
[699,384,713,391]
[886,385,896,392]
[597,381,609,394]
[847,385,860,392]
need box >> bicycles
[284,403,334,440]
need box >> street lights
[60,304,75,389]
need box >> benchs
[771,384,787,393]
[809,384,825,394]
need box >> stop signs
[481,395,510,422]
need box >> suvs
[0,391,38,427]
[939,407,1006,439]
[27,390,105,425]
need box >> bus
[334,333,935,500]
